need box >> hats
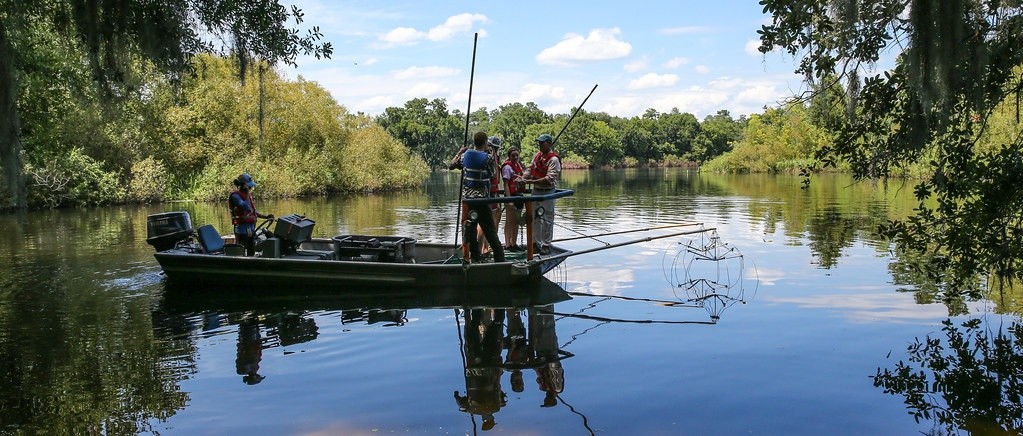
[535,134,553,142]
[238,174,257,187]
[488,135,502,149]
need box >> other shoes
[532,242,542,255]
[503,245,517,252]
[539,245,551,255]
[513,244,524,251]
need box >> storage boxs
[274,214,315,242]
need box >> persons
[236,318,266,386]
[452,305,565,430]
[228,174,273,256]
[447,132,562,262]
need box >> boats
[154,280,575,371]
[145,188,715,288]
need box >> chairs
[197,224,228,255]
